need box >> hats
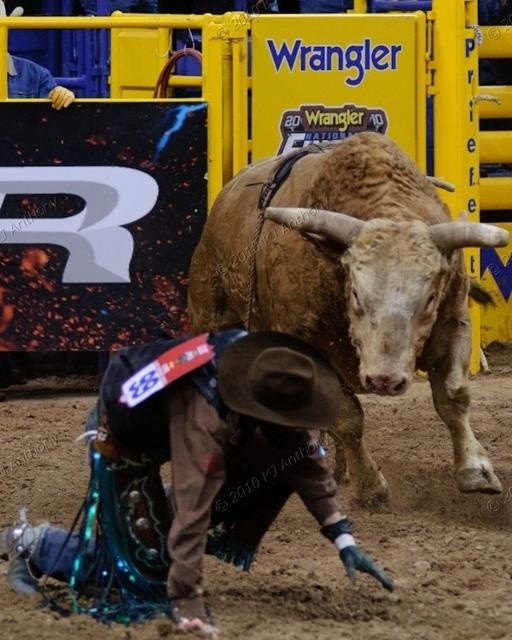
[216,330,343,429]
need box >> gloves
[339,545,395,593]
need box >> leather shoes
[0,519,42,597]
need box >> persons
[1,1,76,111]
[0,329,395,639]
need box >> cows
[186,130,511,508]
